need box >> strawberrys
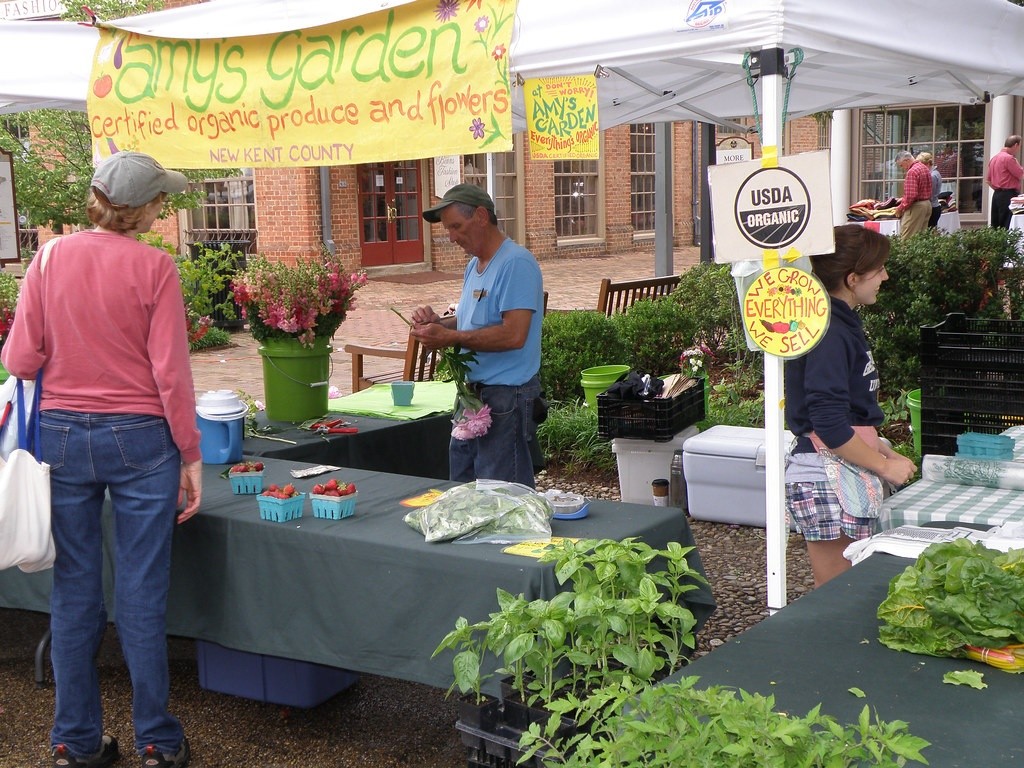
[313,479,356,497]
[232,461,264,473]
[263,483,300,500]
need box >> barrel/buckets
[906,389,921,456]
[581,365,630,413]
[257,337,334,421]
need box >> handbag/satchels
[1,370,56,573]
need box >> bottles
[670,451,690,518]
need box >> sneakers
[141,735,191,768]
[51,733,119,768]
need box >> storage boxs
[921,314,1024,456]
[256,492,307,522]
[596,377,890,531]
[196,639,359,708]
[309,490,358,520]
[226,464,265,494]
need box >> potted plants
[431,536,711,753]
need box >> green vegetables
[403,482,553,542]
[876,539,1024,689]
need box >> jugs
[195,390,249,464]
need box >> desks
[242,409,548,480]
[845,210,961,236]
[620,521,1023,768]
[0,454,718,702]
[880,423,1024,533]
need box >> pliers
[312,419,360,437]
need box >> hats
[90,149,189,210]
[421,183,494,223]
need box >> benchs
[545,274,680,318]
[346,290,550,395]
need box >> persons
[893,150,942,241]
[784,223,918,589]
[1,150,204,768]
[410,182,548,491]
[985,135,1024,233]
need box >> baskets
[954,434,1014,463]
[257,492,308,522]
[228,462,264,494]
[309,490,359,520]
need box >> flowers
[679,341,718,379]
[392,307,492,441]
[228,242,369,350]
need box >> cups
[652,479,669,497]
[653,495,669,507]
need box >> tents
[80,3,1024,621]
